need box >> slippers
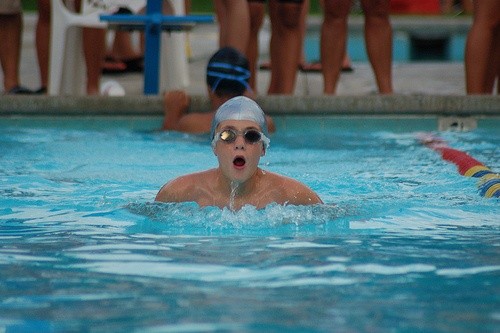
[301,54,353,72]
[259,57,306,69]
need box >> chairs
[48,0,189,96]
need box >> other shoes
[99,54,127,74]
[120,55,144,73]
[7,85,46,95]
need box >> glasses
[211,130,270,147]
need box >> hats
[210,96,270,142]
[206,47,250,97]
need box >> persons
[154,96,323,210]
[0,0,500,134]
